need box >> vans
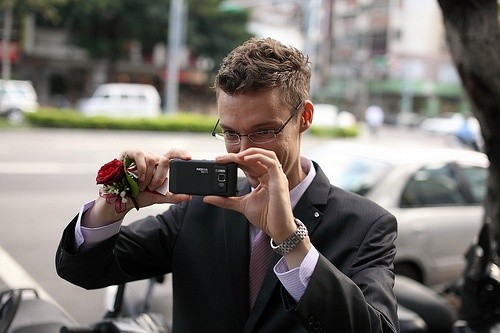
[82,82,161,120]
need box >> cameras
[168,158,237,197]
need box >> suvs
[2,80,39,129]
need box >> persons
[55,38,401,333]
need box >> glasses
[212,100,303,144]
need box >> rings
[279,164,283,169]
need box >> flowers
[96,155,140,211]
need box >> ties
[250,232,274,310]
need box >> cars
[364,145,490,289]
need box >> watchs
[270,217,308,255]
